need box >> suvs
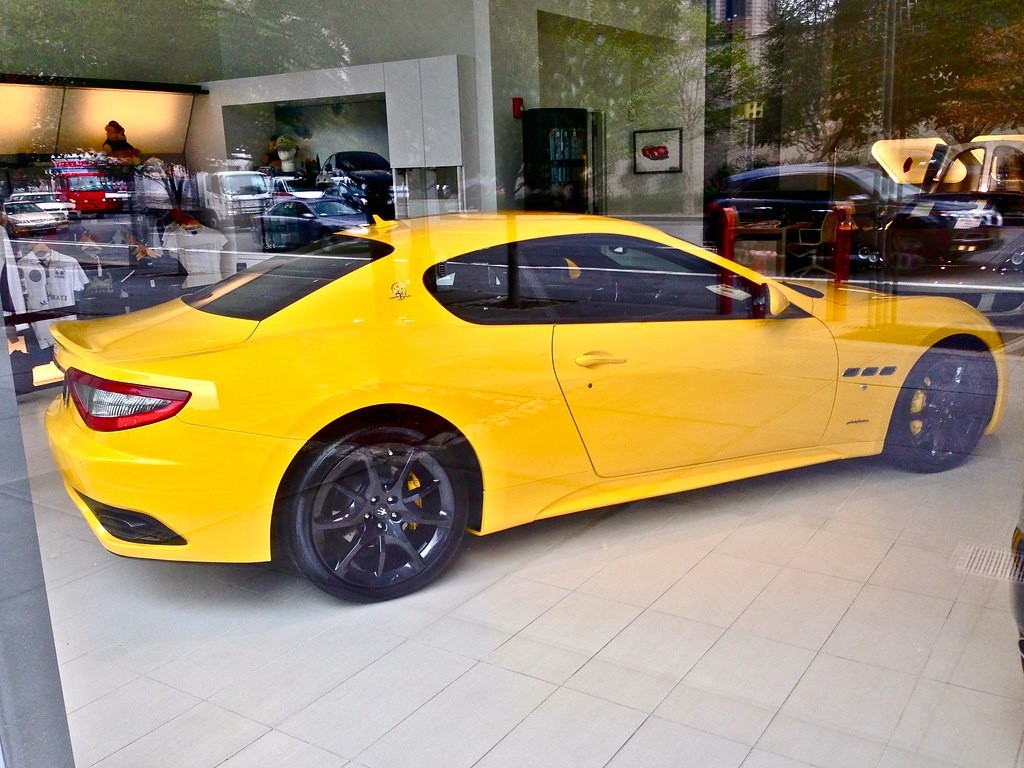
[9,191,83,225]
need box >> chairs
[789,212,840,279]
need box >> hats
[274,136,300,152]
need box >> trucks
[198,170,273,231]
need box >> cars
[44,205,1009,604]
[315,150,393,200]
[0,199,60,241]
[265,170,393,222]
[258,196,373,253]
[707,159,1005,276]
[870,131,1024,227]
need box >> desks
[737,220,810,277]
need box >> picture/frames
[633,126,683,174]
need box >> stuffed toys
[102,120,142,166]
[261,135,281,169]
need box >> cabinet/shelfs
[522,107,589,212]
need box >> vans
[46,152,123,220]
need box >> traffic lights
[755,100,763,118]
[746,100,757,121]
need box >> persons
[0,215,19,255]
[38,180,47,202]
[273,136,300,172]
